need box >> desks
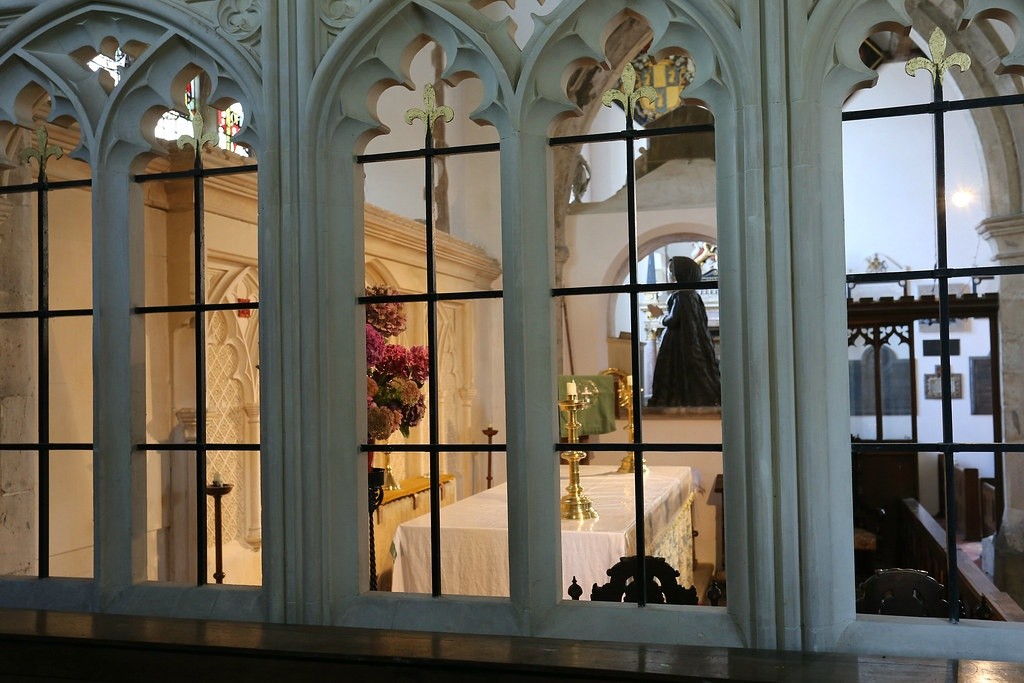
[390,466,695,601]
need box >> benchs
[935,455,997,543]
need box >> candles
[213,472,221,483]
[627,375,633,386]
[566,381,576,396]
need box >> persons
[648,256,721,407]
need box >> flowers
[362,285,431,441]
